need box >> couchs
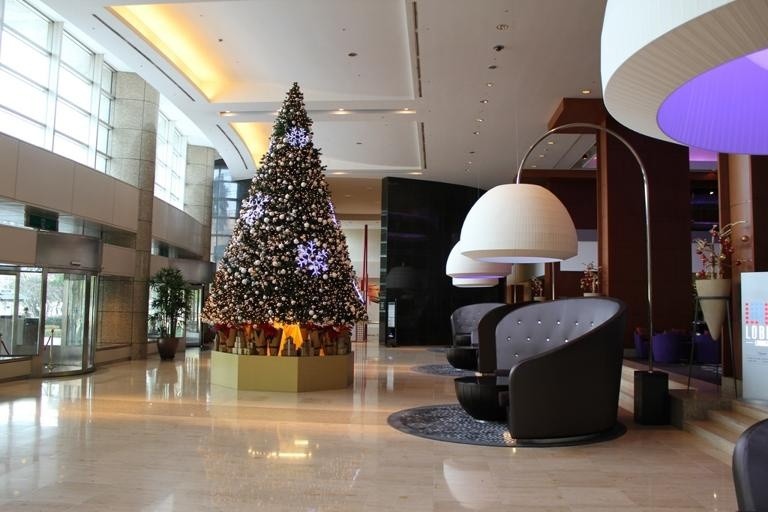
[728,414,768,511]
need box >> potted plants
[143,264,195,361]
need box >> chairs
[633,322,724,366]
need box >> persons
[23,307,34,318]
